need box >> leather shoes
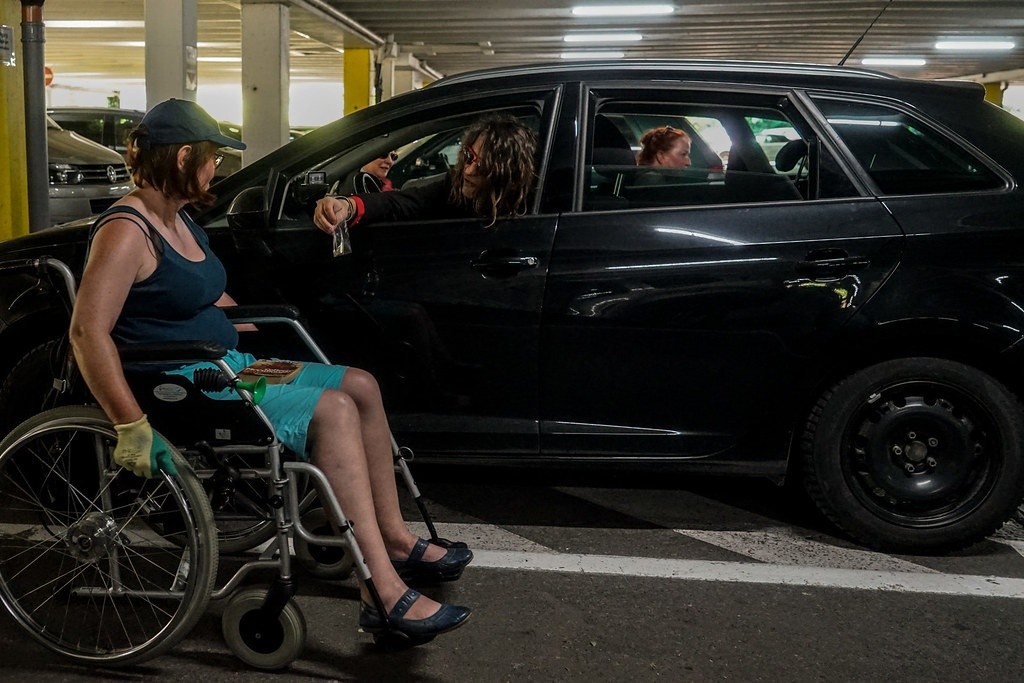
[391,538,473,574]
[358,588,472,632]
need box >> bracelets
[330,194,354,221]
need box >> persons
[66,92,473,640]
[314,106,538,238]
[634,127,694,170]
[359,148,397,192]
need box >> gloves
[113,414,177,479]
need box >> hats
[137,97,247,150]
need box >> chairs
[719,133,809,205]
[585,112,640,214]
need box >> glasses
[213,152,225,170]
[463,145,484,175]
[380,152,398,161]
[654,125,676,143]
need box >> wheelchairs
[0,249,468,673]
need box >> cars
[0,56,1023,568]
[45,109,135,229]
[49,103,322,198]
